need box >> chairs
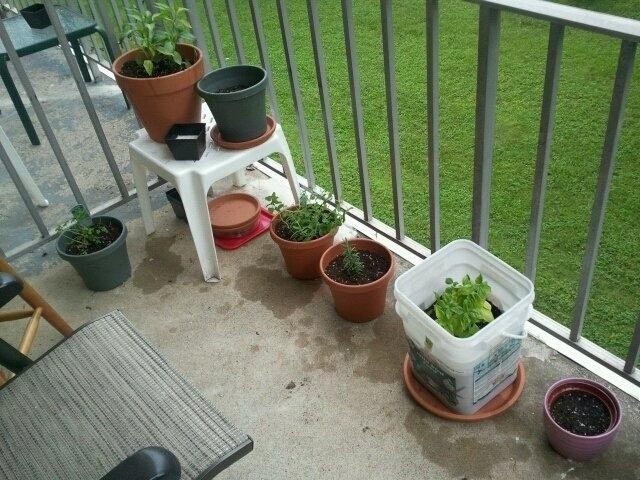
[0,306,256,479]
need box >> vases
[539,377,622,463]
[165,64,274,164]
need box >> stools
[128,103,303,285]
[1,5,131,147]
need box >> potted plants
[320,235,396,324]
[266,185,353,282]
[55,201,139,290]
[109,1,204,144]
